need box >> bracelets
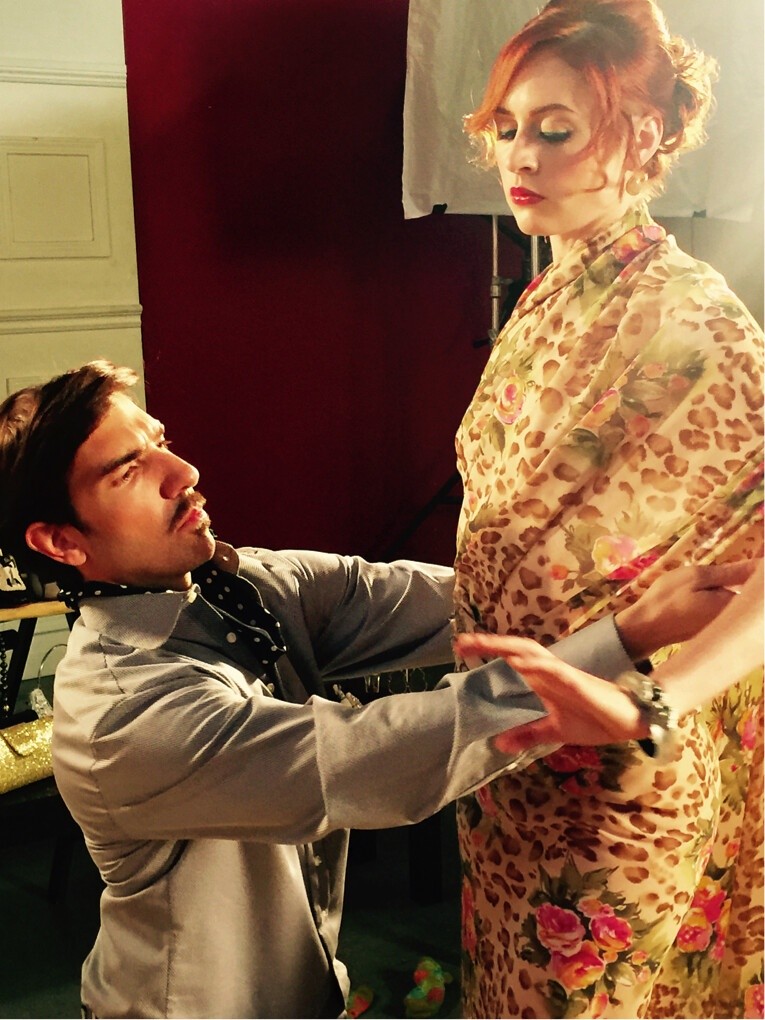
[613,669,677,766]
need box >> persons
[0,360,760,1020]
[452,0,765,1020]
